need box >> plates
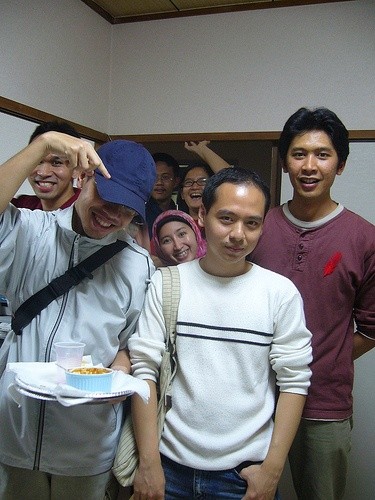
[15,364,136,401]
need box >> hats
[94,140,157,224]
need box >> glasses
[154,175,174,184]
[183,178,207,186]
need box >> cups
[56,342,86,367]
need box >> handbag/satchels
[112,352,178,486]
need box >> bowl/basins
[66,367,115,394]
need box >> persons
[251,106,375,500]
[0,132,154,500]
[128,166,312,500]
[7,122,238,267]
